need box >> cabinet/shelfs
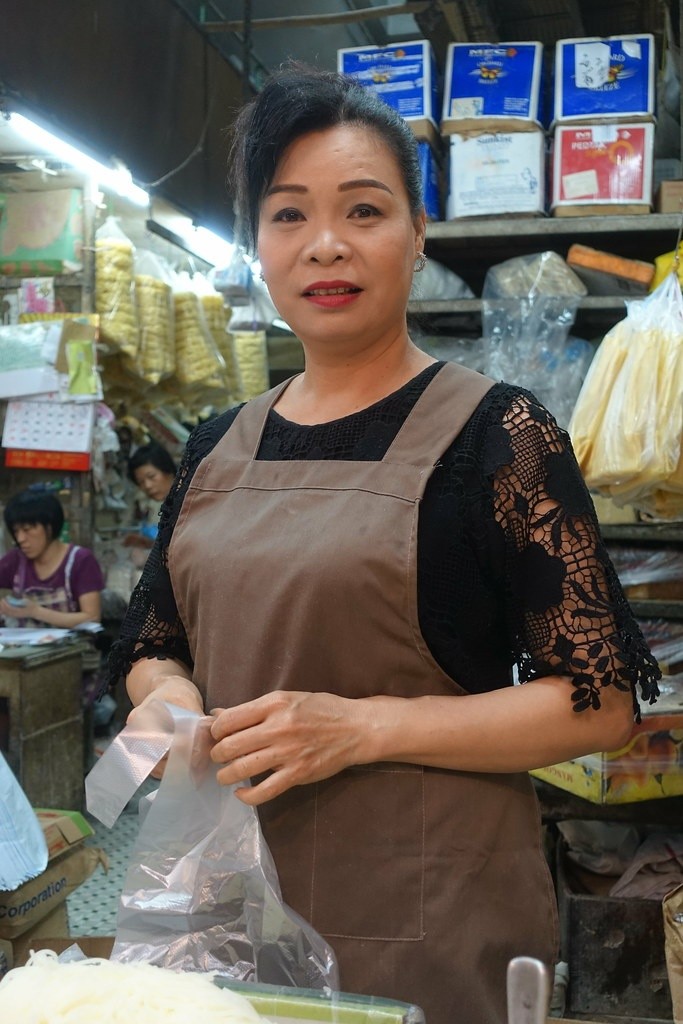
[225,213,683,830]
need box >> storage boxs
[0,170,92,278]
[0,807,110,977]
[337,34,683,220]
[527,663,683,804]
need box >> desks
[1,637,93,810]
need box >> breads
[93,241,270,411]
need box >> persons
[127,444,178,502]
[0,488,107,778]
[116,58,665,1023]
